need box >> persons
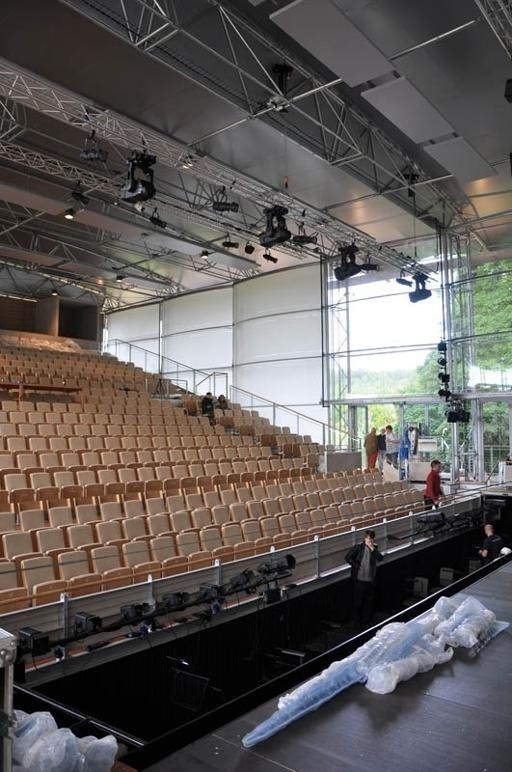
[378,429,386,458]
[384,426,401,469]
[344,528,383,631]
[216,395,228,409]
[424,459,444,510]
[202,392,215,425]
[478,522,502,565]
[364,428,378,469]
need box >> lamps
[62,127,437,303]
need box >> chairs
[0,328,437,617]
